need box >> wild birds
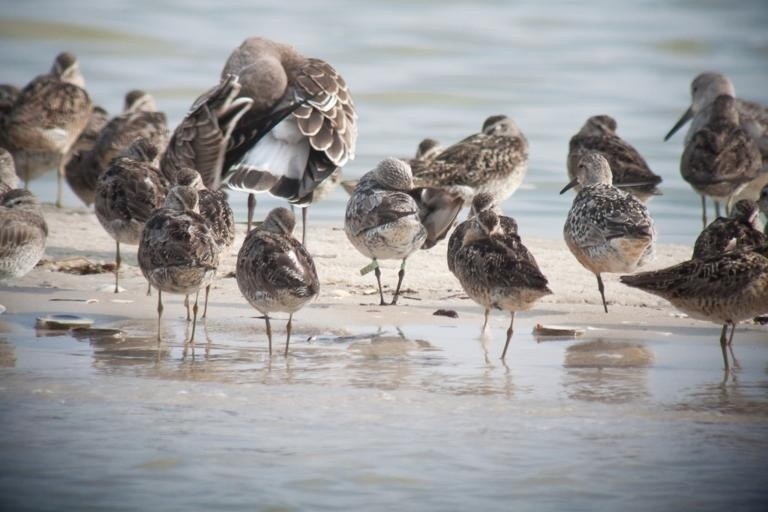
[681,93,762,229]
[566,114,663,205]
[0,150,50,317]
[664,70,768,218]
[0,42,170,212]
[618,242,768,345]
[559,152,656,315]
[341,113,529,305]
[158,36,359,255]
[691,199,768,260]
[165,170,240,319]
[455,208,555,336]
[138,187,216,348]
[236,207,320,361]
[95,140,168,297]
